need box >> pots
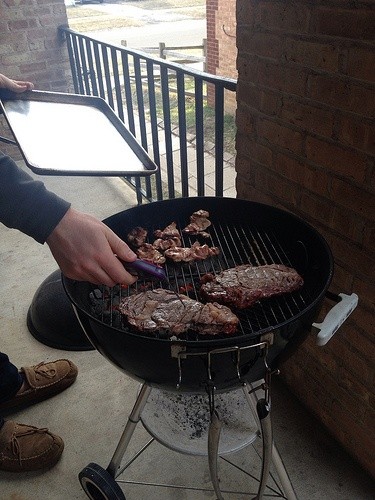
[60,196,334,393]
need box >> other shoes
[0,420,64,472]
[1,358,78,418]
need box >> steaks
[118,287,241,337]
[199,263,304,309]
[127,209,219,269]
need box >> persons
[0,70,138,474]
